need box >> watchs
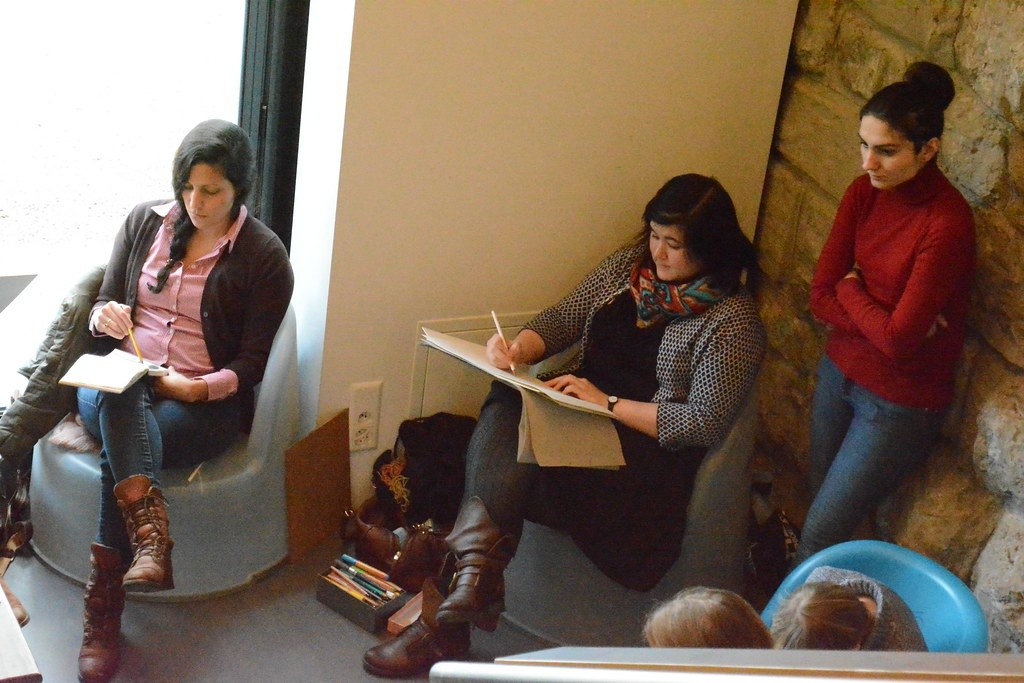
[607,395,618,411]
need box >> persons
[770,564,931,653]
[642,586,776,649]
[362,174,765,677]
[75,117,293,683]
[794,58,978,572]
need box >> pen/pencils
[120,305,144,363]
[491,310,516,377]
[322,553,403,609]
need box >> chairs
[758,541,990,655]
[26,302,302,605]
[496,392,761,650]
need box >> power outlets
[349,381,381,451]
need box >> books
[59,349,170,395]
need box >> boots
[114,474,174,594]
[363,576,472,679]
[436,495,517,632]
[77,541,124,682]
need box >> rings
[105,319,111,327]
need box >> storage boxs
[387,590,427,636]
[316,558,406,635]
